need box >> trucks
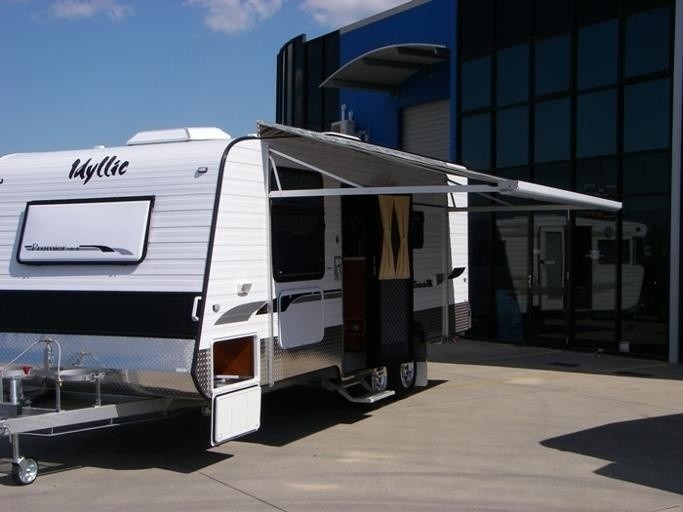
[1,119,625,488]
[494,214,649,318]
